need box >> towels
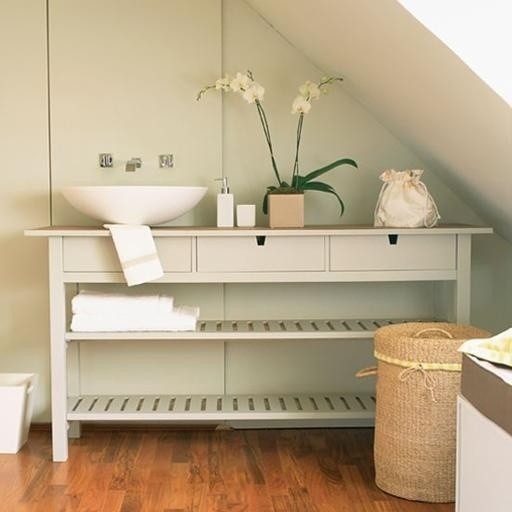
[101,222,165,288]
[70,306,202,332]
[68,290,176,313]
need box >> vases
[266,193,306,228]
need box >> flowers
[193,67,359,219]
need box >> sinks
[60,184,207,227]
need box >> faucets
[125,157,142,173]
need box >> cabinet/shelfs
[22,222,496,463]
[453,352,512,512]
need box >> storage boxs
[0,373,40,453]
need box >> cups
[235,203,257,228]
